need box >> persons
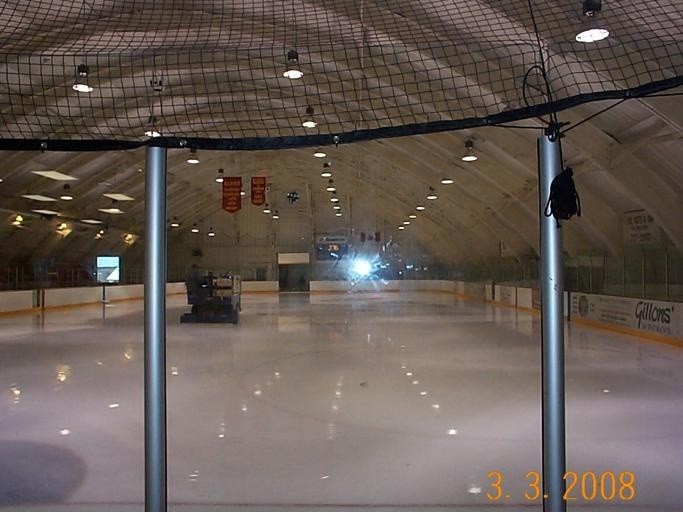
[207,271,218,297]
[185,264,206,313]
[298,274,306,291]
